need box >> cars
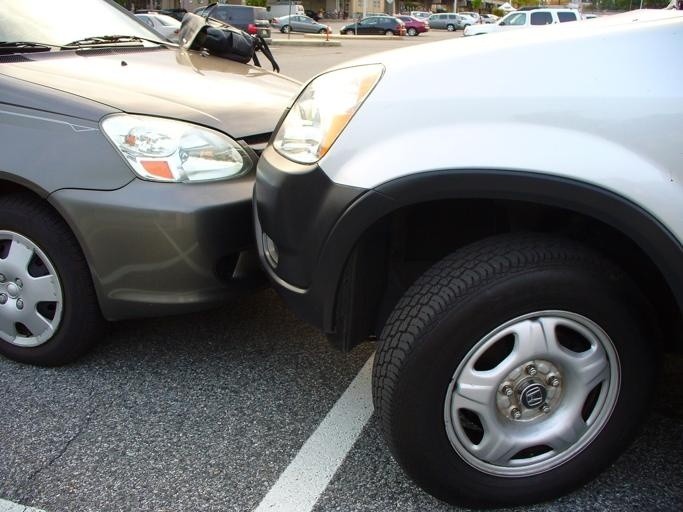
[271,13,333,35]
[339,15,407,37]
[252,7,683,509]
[397,7,596,38]
[134,5,273,47]
[0,0,304,367]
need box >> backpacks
[178,2,279,74]
